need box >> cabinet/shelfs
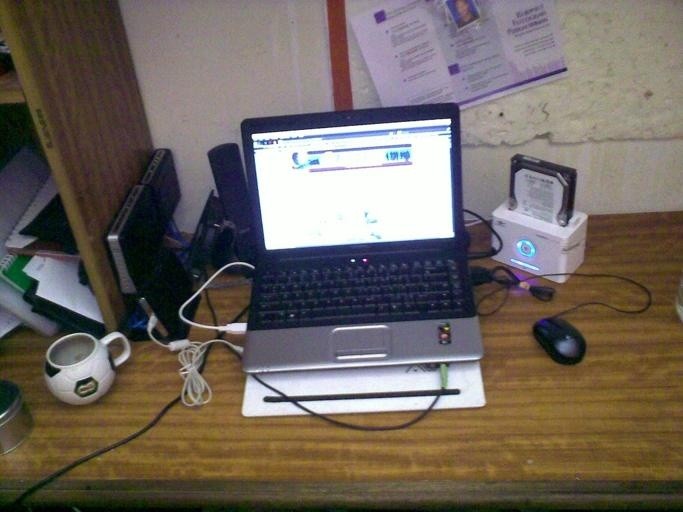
[0,3,153,336]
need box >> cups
[44,332,132,406]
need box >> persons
[453,0,479,29]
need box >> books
[0,147,106,341]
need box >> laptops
[241,102,483,373]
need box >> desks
[1,211,683,512]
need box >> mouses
[534,316,586,365]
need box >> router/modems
[140,268,191,336]
